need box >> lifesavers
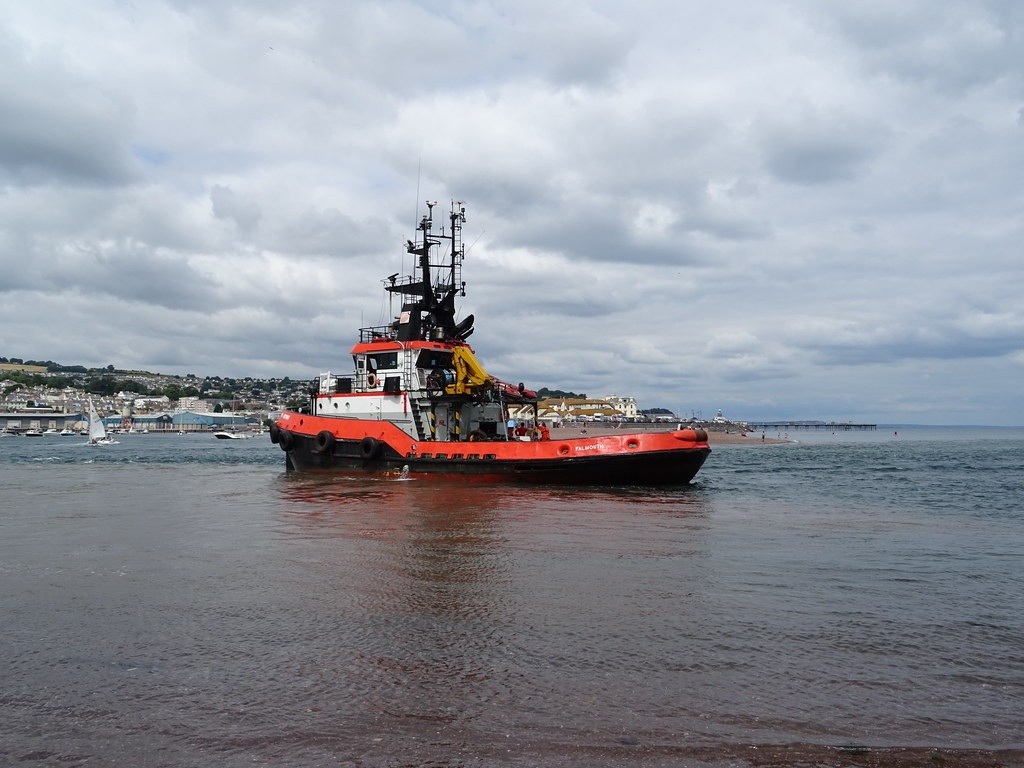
[367,373,376,388]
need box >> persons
[537,422,550,441]
[724,428,898,443]
[515,422,528,436]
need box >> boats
[262,196,714,490]
[0,421,265,442]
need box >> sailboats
[87,395,120,446]
